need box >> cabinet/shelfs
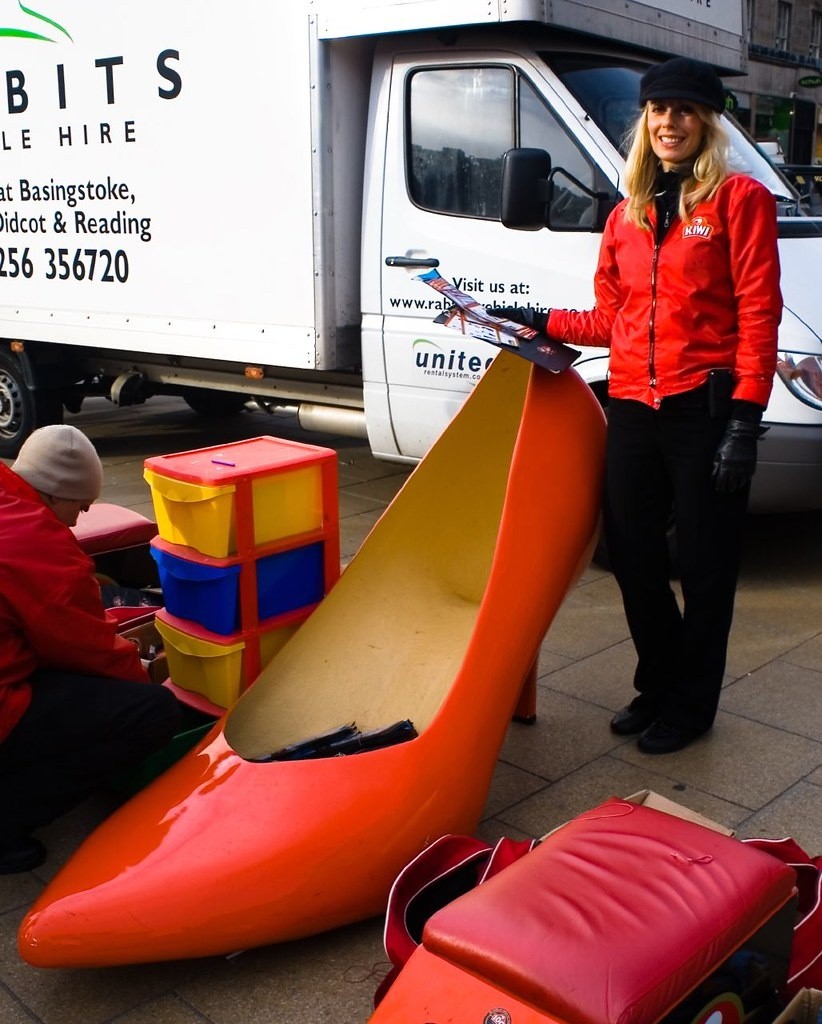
[141,434,341,753]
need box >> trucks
[0,0,822,570]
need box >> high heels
[20,338,609,969]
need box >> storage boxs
[142,465,325,708]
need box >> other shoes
[611,693,681,734]
[638,713,713,753]
[0,836,48,875]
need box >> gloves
[713,421,770,494]
[486,308,549,332]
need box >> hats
[639,57,725,114]
[11,424,103,500]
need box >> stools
[414,796,799,1024]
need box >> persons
[0,422,183,869]
[488,60,783,757]
